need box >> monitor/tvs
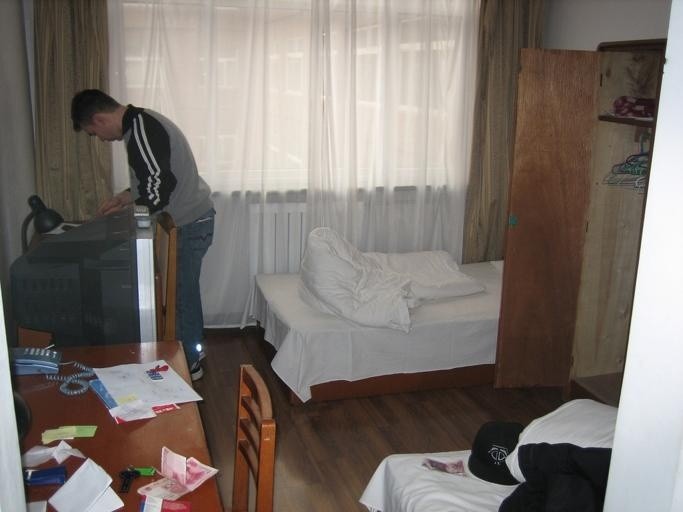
[9,206,161,343]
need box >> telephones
[8,347,63,375]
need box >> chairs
[155,211,177,340]
[233,364,276,512]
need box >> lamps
[21,195,64,254]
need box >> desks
[10,340,225,512]
[28,220,164,341]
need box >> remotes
[136,217,150,229]
[134,206,148,215]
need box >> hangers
[601,132,652,193]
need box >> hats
[468,422,521,485]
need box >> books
[85,359,201,423]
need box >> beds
[387,450,524,512]
[255,260,504,407]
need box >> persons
[70,87,216,382]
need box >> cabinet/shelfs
[494,38,668,403]
